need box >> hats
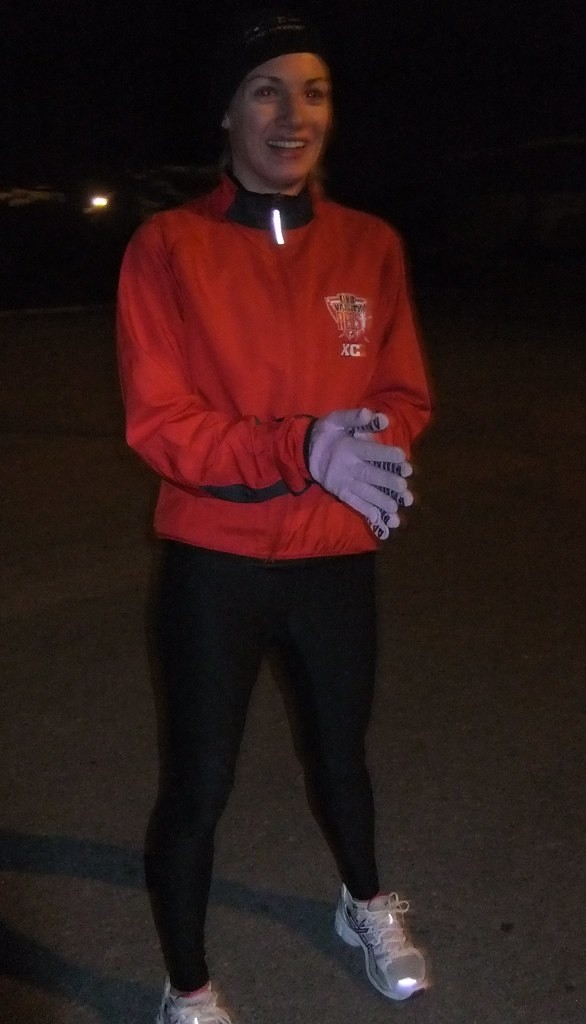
[214,16,335,156]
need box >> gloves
[309,407,415,540]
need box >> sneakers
[334,882,429,1002]
[159,976,234,1024]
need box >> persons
[117,8,433,1024]
